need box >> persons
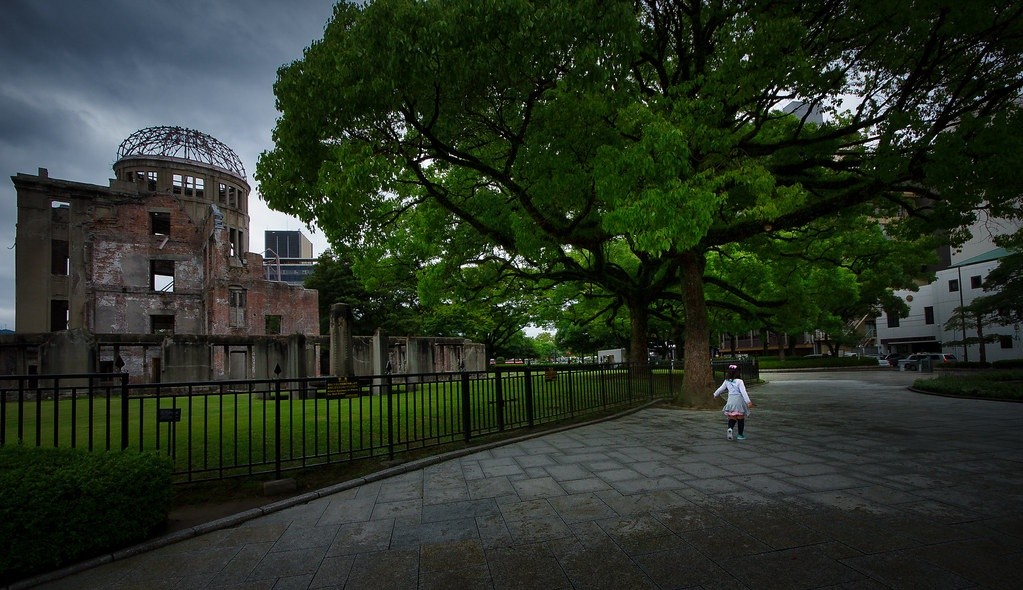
[713,365,753,441]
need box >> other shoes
[737,435,747,440]
[727,429,733,440]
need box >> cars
[865,354,890,367]
[886,353,907,367]
[898,355,917,369]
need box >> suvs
[908,353,958,371]
